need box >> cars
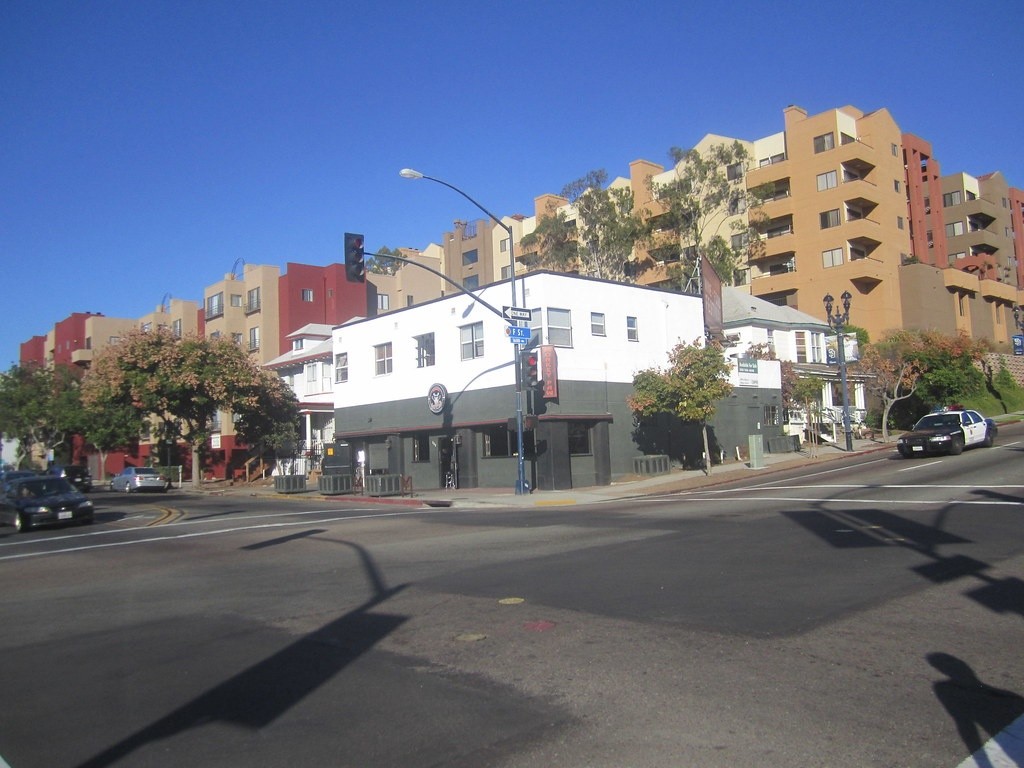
[110,467,167,493]
[0,465,93,532]
[897,405,998,458]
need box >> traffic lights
[344,232,366,283]
[531,380,546,415]
[521,352,538,388]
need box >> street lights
[398,169,531,496]
[823,290,853,452]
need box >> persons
[21,484,31,497]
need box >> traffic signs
[502,307,530,320]
